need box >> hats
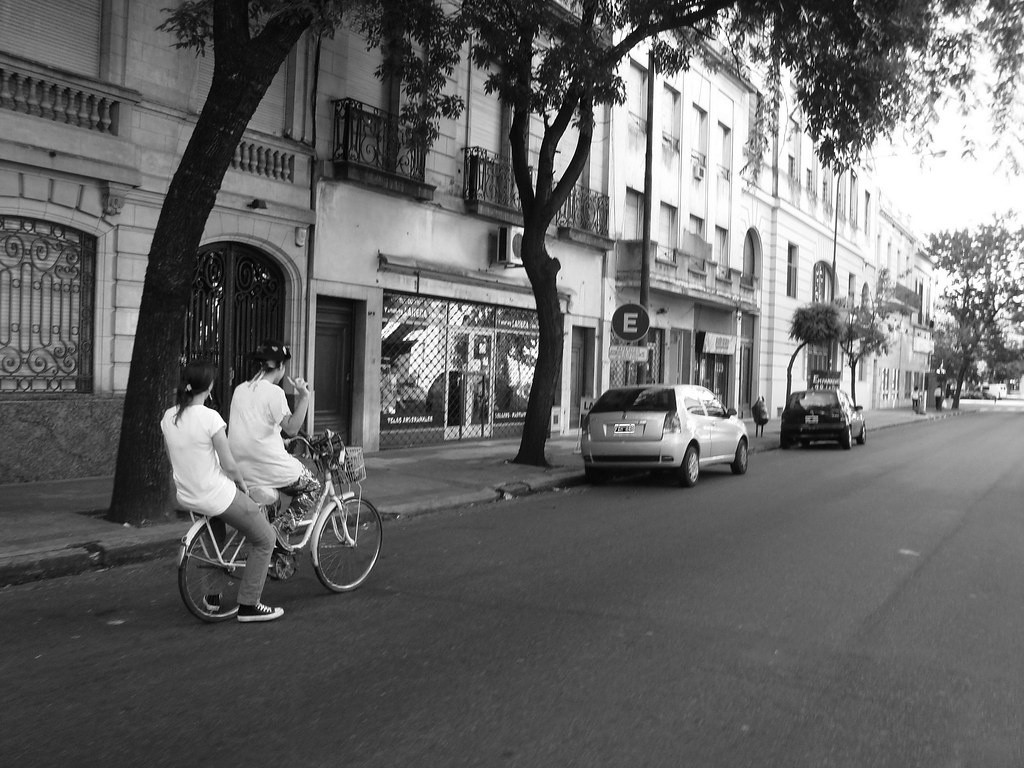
[254,339,287,370]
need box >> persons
[160,362,283,621]
[934,385,945,412]
[226,338,321,551]
[911,386,919,410]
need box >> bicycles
[177,427,383,623]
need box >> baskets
[330,446,367,484]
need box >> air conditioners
[496,225,525,267]
[694,164,705,180]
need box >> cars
[961,383,1006,400]
[581,384,750,487]
[424,371,489,416]
[779,391,867,450]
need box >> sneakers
[236,604,284,622]
[269,518,294,553]
[202,592,223,610]
